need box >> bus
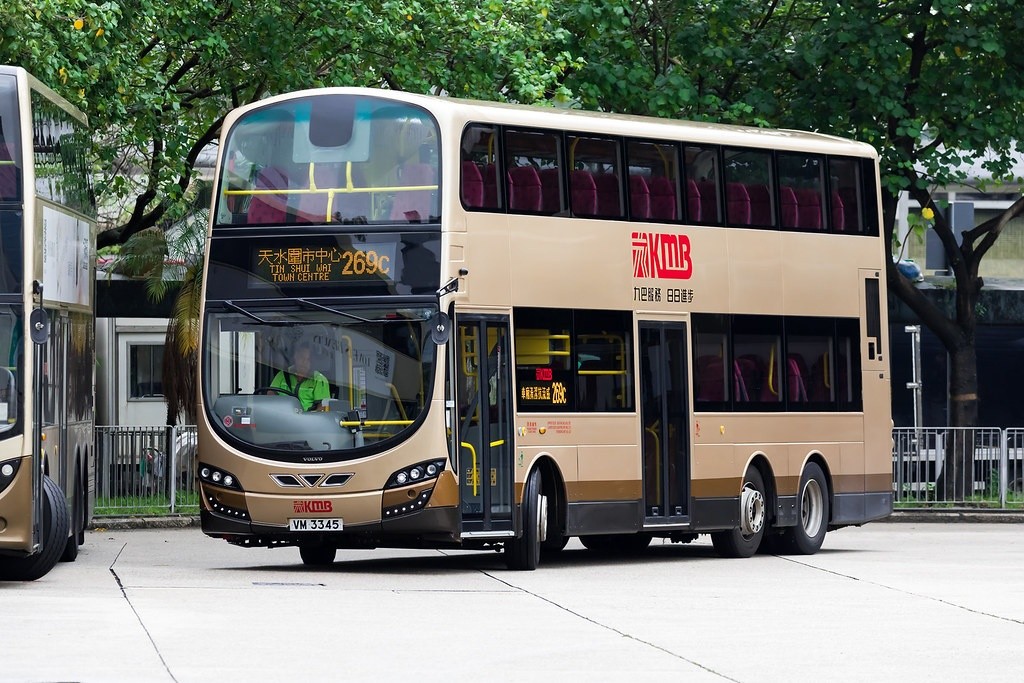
[1,63,99,581]
[196,86,894,571]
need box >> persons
[267,340,332,413]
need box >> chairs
[461,160,844,233]
[297,166,336,222]
[695,353,846,403]
[387,162,432,219]
[247,167,288,224]
